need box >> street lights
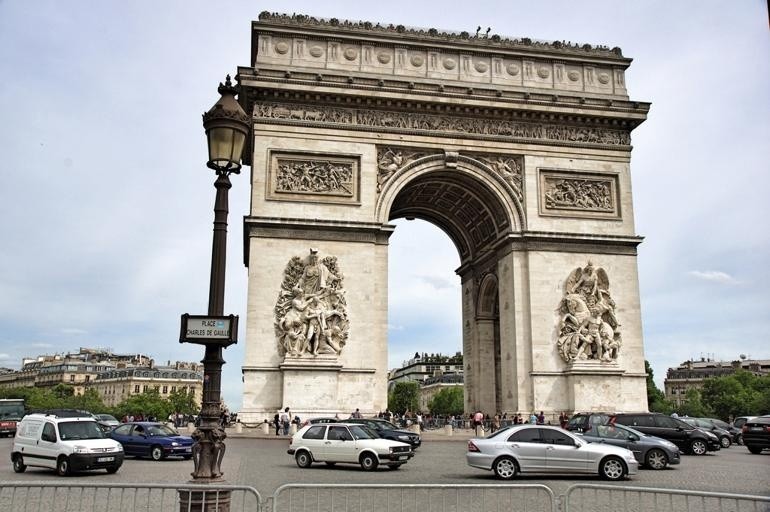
[175,73,249,512]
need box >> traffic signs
[179,313,239,346]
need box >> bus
[0,398,26,438]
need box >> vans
[605,411,722,456]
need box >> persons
[670,411,678,418]
[284,253,341,356]
[278,161,350,192]
[571,263,621,361]
[729,414,734,426]
[546,180,611,208]
[683,413,688,417]
[480,156,523,200]
[378,148,419,190]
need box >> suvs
[565,411,609,433]
[741,415,770,454]
[10,410,124,476]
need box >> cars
[286,423,415,472]
[574,422,682,470]
[303,417,337,431]
[73,410,193,438]
[337,418,422,451]
[675,417,733,448]
[729,415,758,446]
[705,418,745,445]
[105,422,194,461]
[464,425,639,481]
